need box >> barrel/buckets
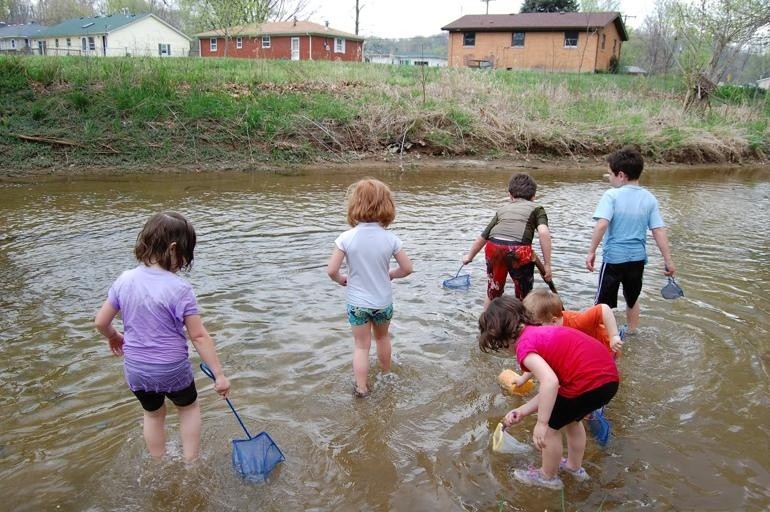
[497,369,534,396]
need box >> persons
[463,174,552,311]
[94,211,230,461]
[586,147,675,331]
[478,296,619,491]
[511,288,623,448]
[327,180,412,397]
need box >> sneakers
[513,464,564,491]
[559,457,590,482]
